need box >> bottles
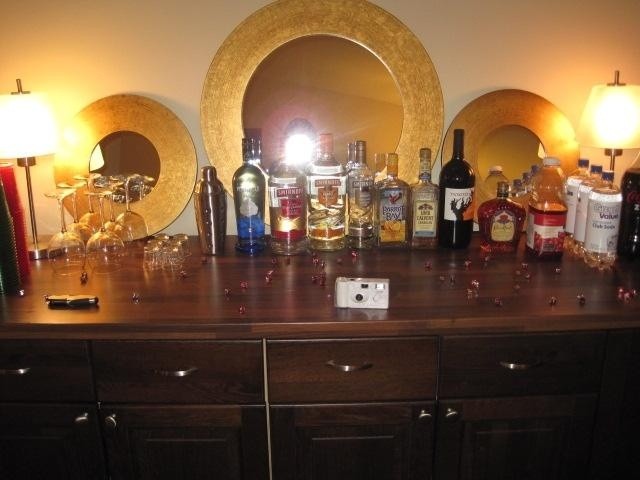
[566,151,640,272]
[348,141,375,253]
[192,166,226,257]
[528,157,567,261]
[483,165,537,197]
[375,129,476,252]
[269,134,347,256]
[232,138,267,256]
[252,135,269,173]
[344,141,355,169]
[478,181,527,256]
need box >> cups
[0,161,29,293]
[143,231,194,273]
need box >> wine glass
[95,174,157,203]
[47,174,147,274]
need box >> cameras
[334,276,389,310]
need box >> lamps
[1,80,65,264]
[577,69,640,188]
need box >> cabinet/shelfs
[1,326,270,480]
[263,324,608,480]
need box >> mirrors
[438,88,581,234]
[54,93,197,239]
[200,0,445,228]
